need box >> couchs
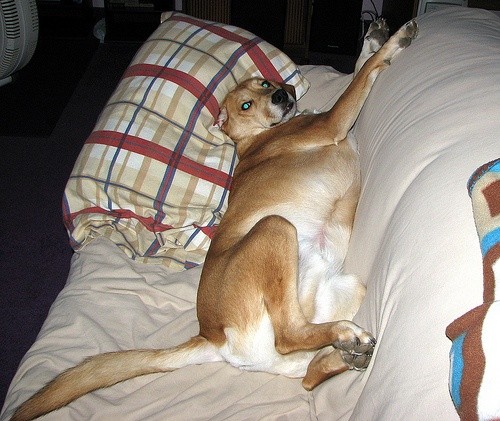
[1,6,500,421]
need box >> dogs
[6,16,420,421]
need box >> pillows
[61,7,313,274]
